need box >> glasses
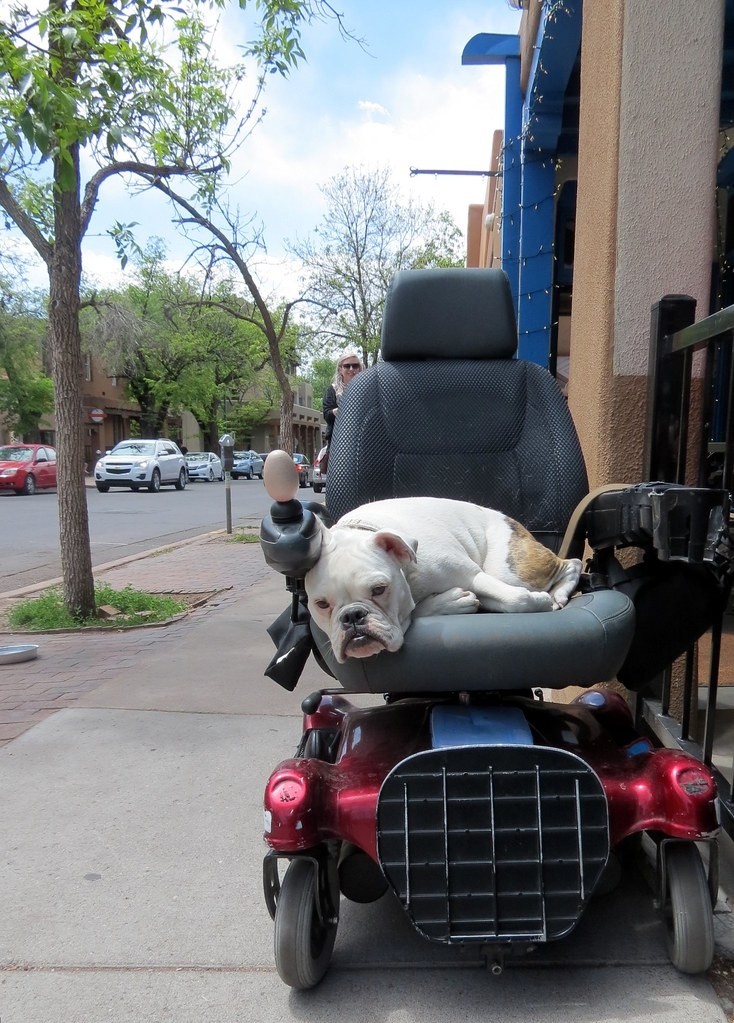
[343,363,359,369]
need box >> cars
[311,445,329,494]
[258,452,268,462]
[223,450,264,480]
[293,453,313,488]
[0,443,58,496]
[183,451,226,482]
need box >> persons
[322,351,365,456]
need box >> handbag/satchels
[317,445,330,474]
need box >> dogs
[303,497,581,664]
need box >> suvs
[94,438,189,493]
